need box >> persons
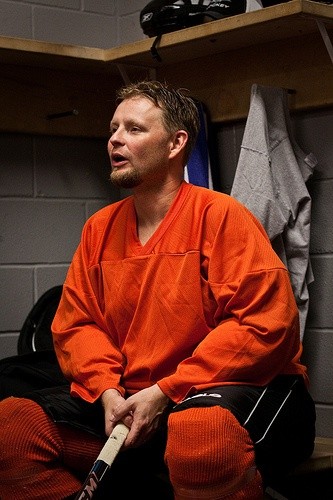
[0,83,310,500]
